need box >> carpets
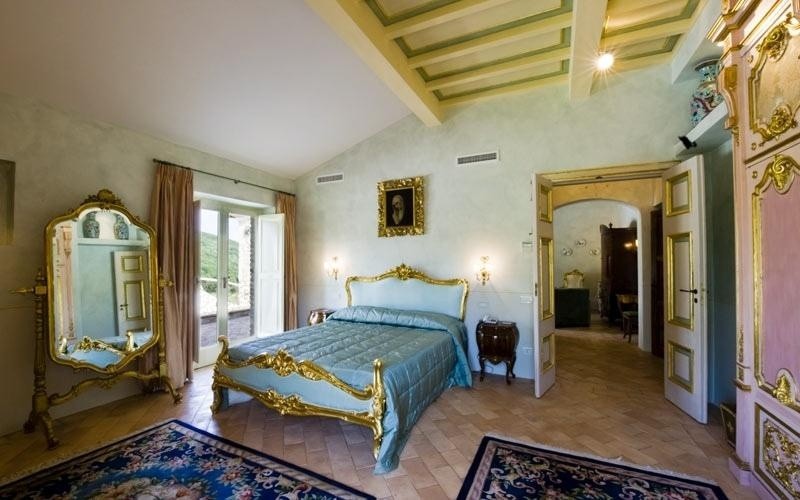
[454,432,729,500]
[0,418,375,500]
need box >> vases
[113,214,129,240]
[83,211,100,238]
[688,58,722,129]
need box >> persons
[388,195,412,226]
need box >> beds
[56,331,152,368]
[209,263,473,476]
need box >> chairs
[622,311,639,343]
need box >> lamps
[476,255,490,287]
[326,256,339,281]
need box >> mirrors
[7,189,183,450]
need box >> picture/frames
[376,176,425,237]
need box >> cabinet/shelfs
[554,287,591,329]
[599,224,637,328]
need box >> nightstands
[475,320,520,384]
[307,308,335,327]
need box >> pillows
[331,303,456,332]
